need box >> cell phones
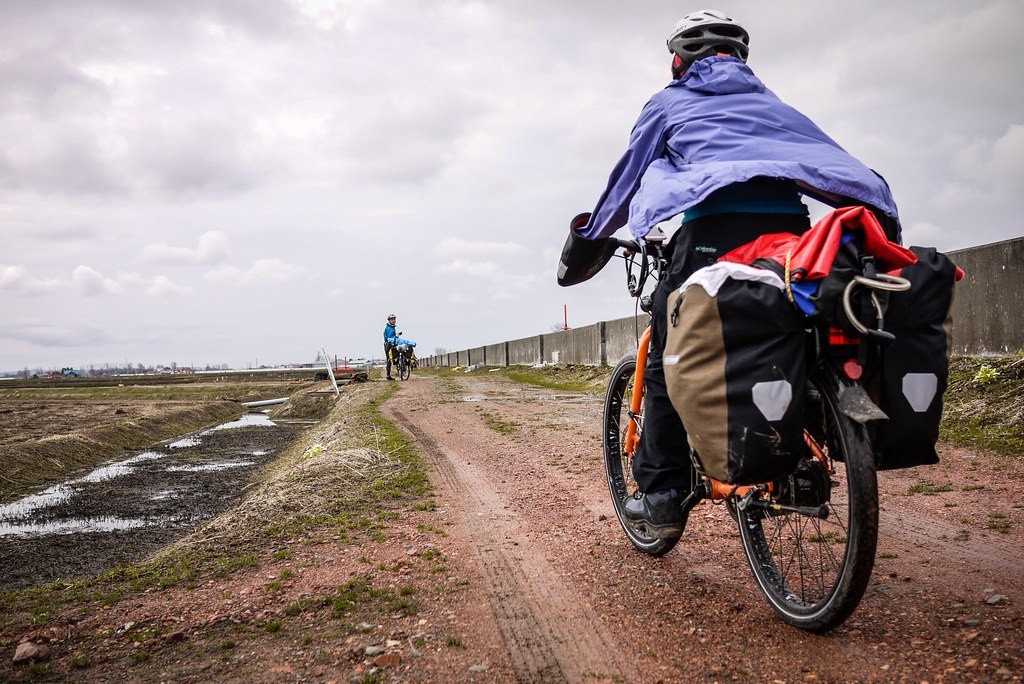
[644,224,667,241]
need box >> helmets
[387,315,396,321]
[666,10,748,61]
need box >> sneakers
[622,486,683,539]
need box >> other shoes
[387,375,395,380]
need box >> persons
[573,9,903,539]
[383,314,396,380]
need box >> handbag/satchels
[663,205,962,496]
[389,344,415,366]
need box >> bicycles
[391,332,411,381]
[558,212,888,633]
